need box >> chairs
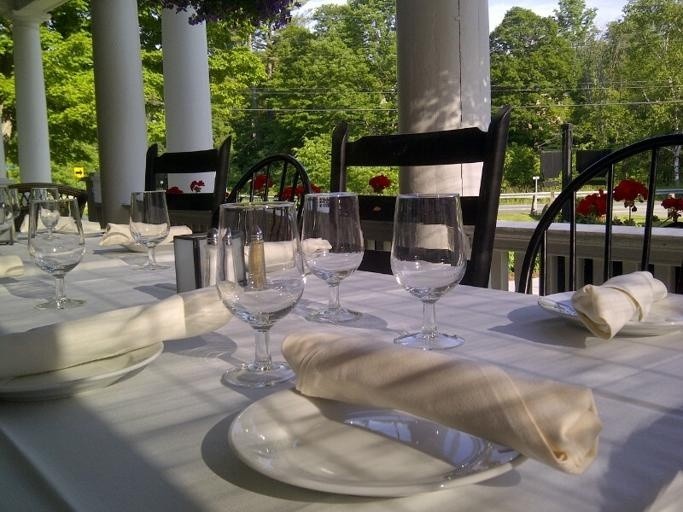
[143,134,232,236]
[10,182,97,220]
[226,155,313,250]
[330,106,520,293]
[517,132,681,295]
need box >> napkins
[204,238,332,276]
[565,270,668,340]
[20,217,105,239]
[98,224,194,254]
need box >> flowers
[189,180,206,192]
[575,186,608,220]
[248,174,274,196]
[278,184,321,202]
[165,185,184,195]
[659,195,681,219]
[366,173,391,195]
[613,177,648,211]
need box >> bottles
[208,226,267,293]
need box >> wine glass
[0,188,60,248]
[30,200,87,309]
[213,201,305,387]
[299,193,362,324]
[129,190,171,270]
[390,193,469,350]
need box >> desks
[0,220,683,512]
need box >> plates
[123,243,175,254]
[228,384,530,500]
[537,290,681,335]
[3,342,165,401]
